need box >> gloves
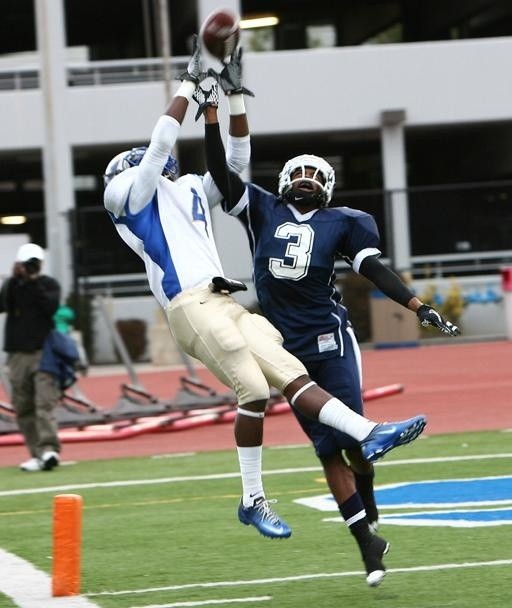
[415,304,459,338]
[179,35,210,84]
[209,47,252,96]
[188,78,220,120]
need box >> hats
[17,243,45,262]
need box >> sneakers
[364,536,390,587]
[21,451,60,471]
[367,513,381,533]
[238,497,293,538]
[362,415,427,465]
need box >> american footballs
[203,12,239,58]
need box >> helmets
[102,145,180,183]
[277,153,337,207]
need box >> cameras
[19,258,40,278]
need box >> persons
[191,65,463,587]
[0,243,62,472]
[100,36,428,539]
